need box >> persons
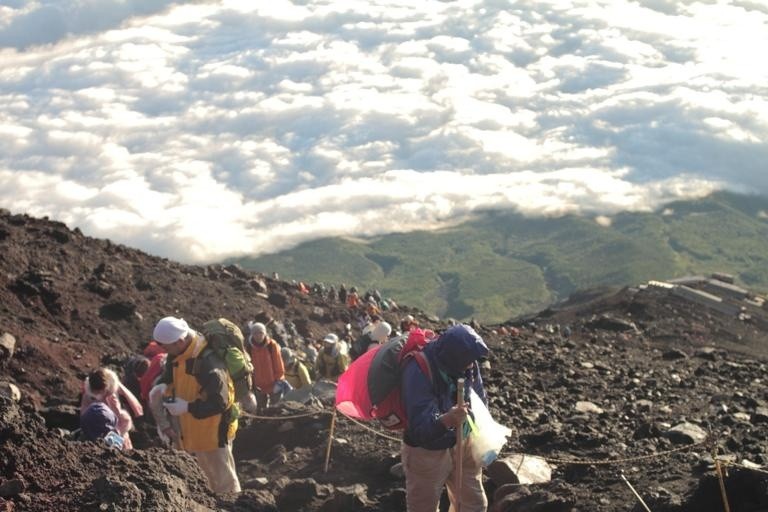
[271,272,382,315]
[81,342,175,449]
[152,316,241,502]
[400,324,489,512]
[362,315,417,355]
[248,322,349,409]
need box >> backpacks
[191,318,252,401]
[368,334,437,430]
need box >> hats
[251,322,266,335]
[373,322,392,338]
[281,347,292,358]
[324,333,338,344]
[153,316,189,345]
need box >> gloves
[163,398,188,416]
[149,383,167,400]
